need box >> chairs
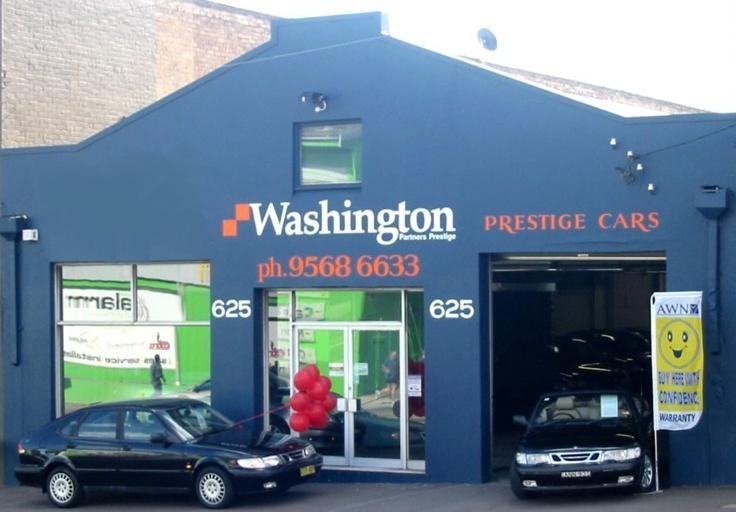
[538,396,624,419]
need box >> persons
[149,354,165,393]
[379,350,400,401]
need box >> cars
[170,376,426,460]
[15,397,323,509]
[504,326,655,499]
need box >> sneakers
[375,390,380,400]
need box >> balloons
[287,362,336,431]
[390,357,426,419]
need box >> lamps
[301,92,327,112]
[610,137,653,191]
